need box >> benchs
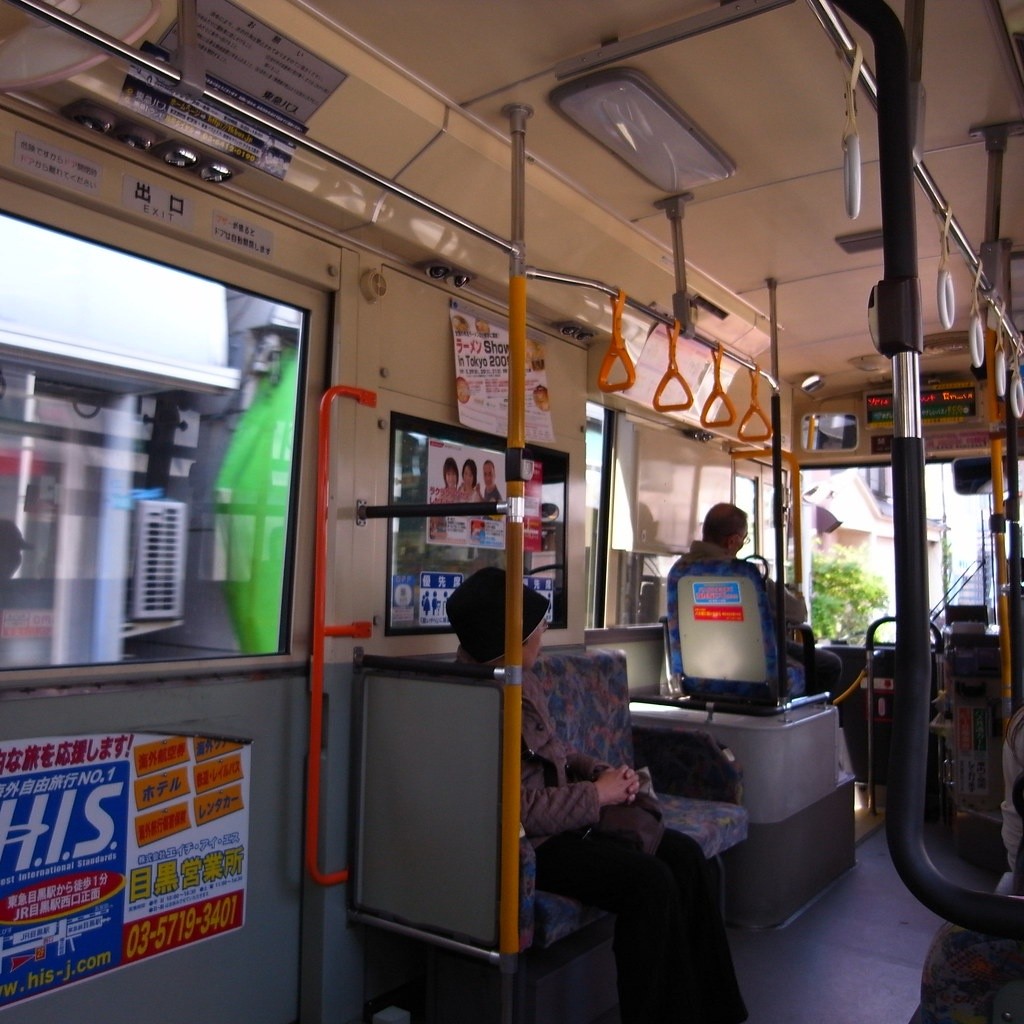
[419,648,750,1024]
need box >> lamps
[549,66,736,193]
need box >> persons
[446,567,752,1024]
[921,703,1024,1024]
[666,504,842,704]
[432,456,503,534]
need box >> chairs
[657,557,829,713]
[866,616,947,825]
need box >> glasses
[733,531,750,545]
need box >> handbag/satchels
[581,790,666,856]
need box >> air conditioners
[132,499,188,620]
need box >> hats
[446,565,551,662]
